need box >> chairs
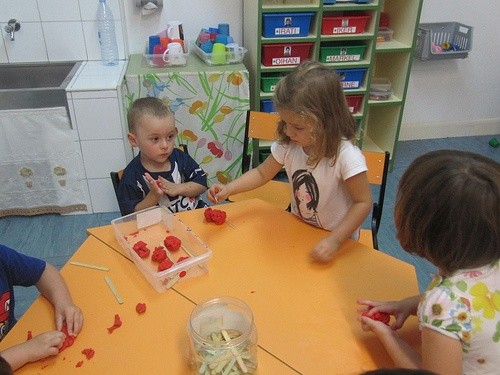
[360,151,393,247]
[224,108,293,211]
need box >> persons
[357,149,500,375]
[0,244,83,375]
[118,97,209,213]
[206,60,373,263]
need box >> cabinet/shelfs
[243,0,426,169]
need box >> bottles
[183,296,258,375]
[95,0,119,66]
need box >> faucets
[4,19,21,41]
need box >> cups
[147,21,188,65]
[195,23,244,65]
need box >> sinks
[0,60,83,110]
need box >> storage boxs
[336,68,367,89]
[322,41,368,62]
[322,13,369,33]
[261,100,280,113]
[262,42,312,64]
[414,22,473,61]
[347,95,362,114]
[263,12,314,35]
[261,74,287,92]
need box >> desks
[0,236,297,375]
[85,198,420,374]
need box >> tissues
[135,0,163,16]
